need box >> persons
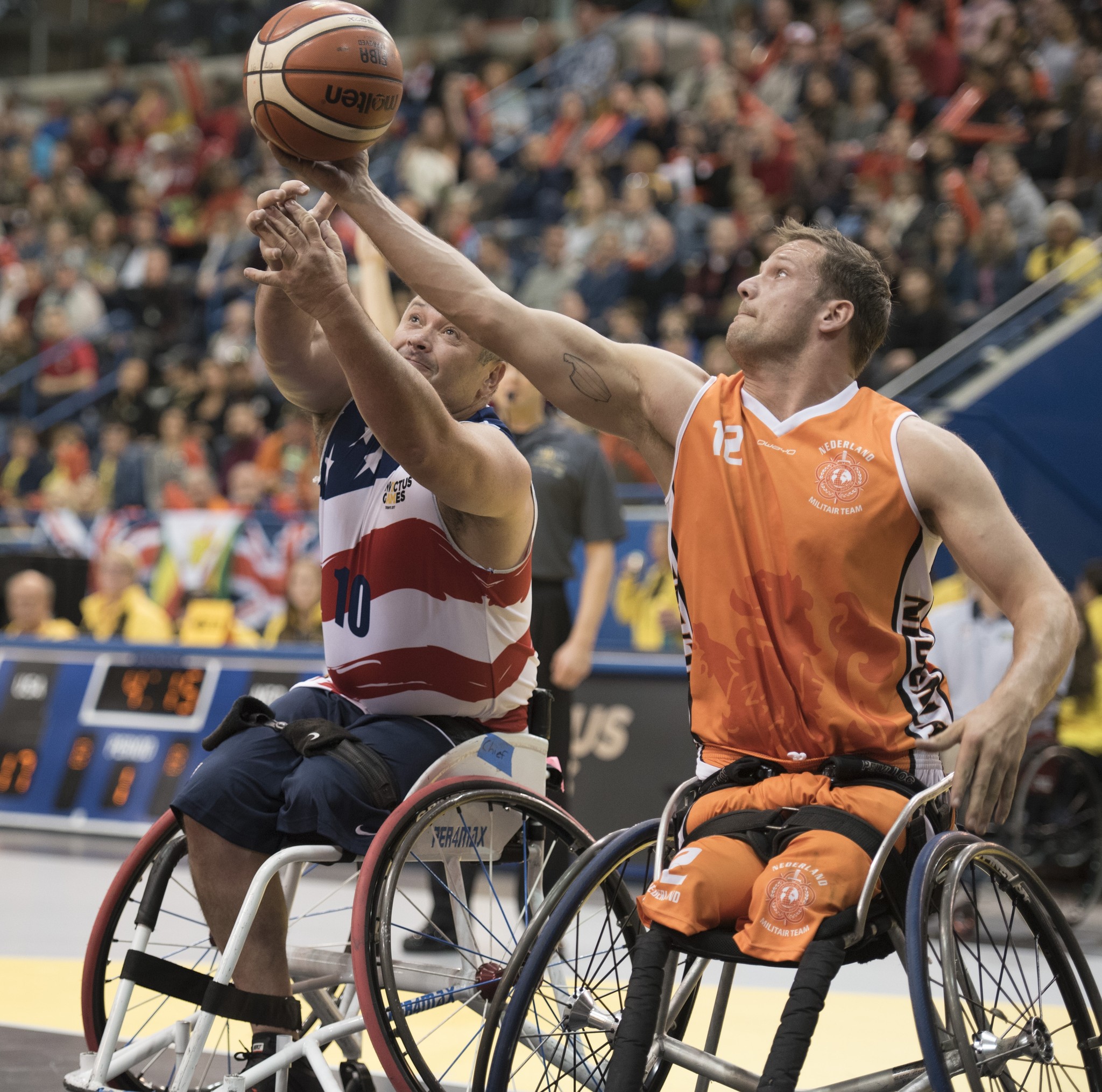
[0,0,1102,961]
[168,178,541,1092]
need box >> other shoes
[954,903,977,938]
[403,910,457,951]
[234,1030,307,1091]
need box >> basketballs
[241,1,405,162]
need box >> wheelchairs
[470,751,1102,1092]
[62,686,646,1092]
